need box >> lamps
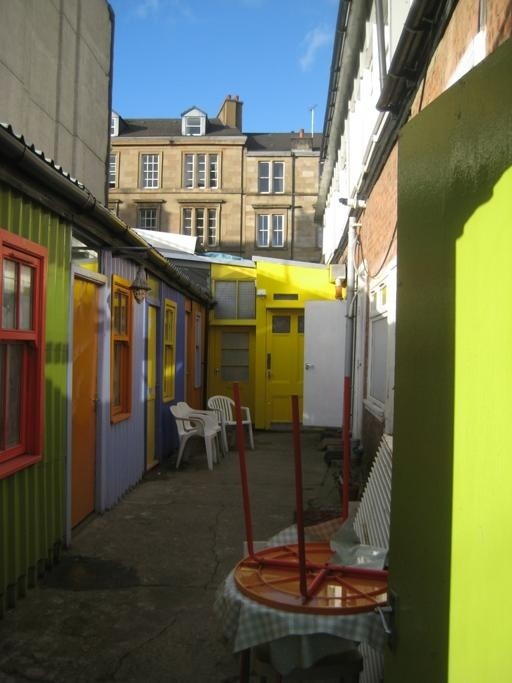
[103,246,156,304]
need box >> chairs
[169,405,216,471]
[207,395,254,449]
[177,402,221,467]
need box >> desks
[226,541,393,681]
[233,377,391,614]
